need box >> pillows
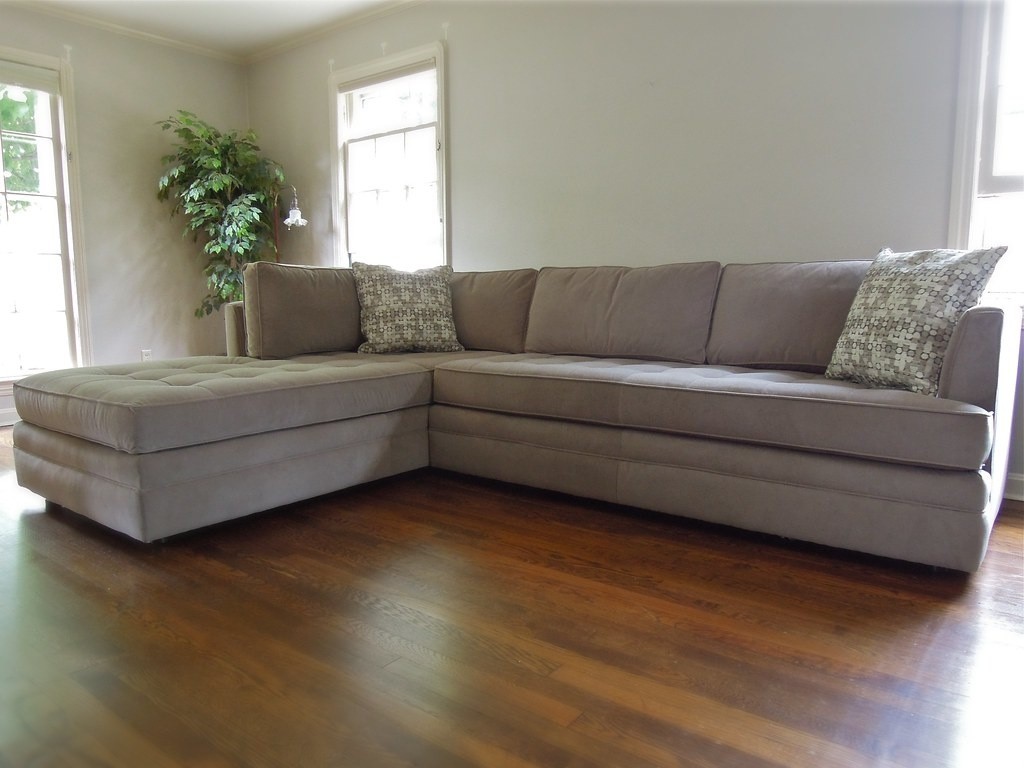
[351,261,465,356]
[524,261,722,365]
[704,258,874,374]
[824,244,1010,396]
[452,268,539,354]
[242,262,364,361]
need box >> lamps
[273,185,307,263]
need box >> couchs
[10,300,1024,574]
